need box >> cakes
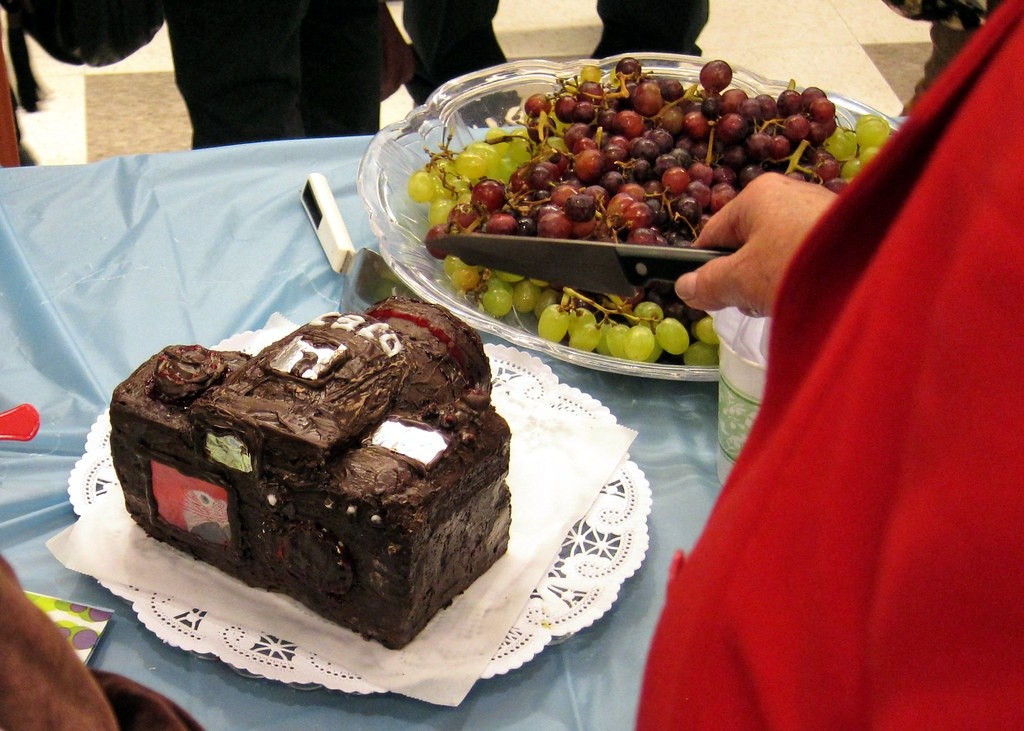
[107,295,513,650]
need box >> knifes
[424,232,736,297]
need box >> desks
[0,125,722,731]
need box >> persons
[882,0,1005,117]
[636,0,1024,731]
[162,0,708,151]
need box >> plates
[357,51,902,381]
[68,328,653,693]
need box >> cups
[717,336,766,484]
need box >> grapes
[406,56,902,368]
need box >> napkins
[42,398,639,709]
